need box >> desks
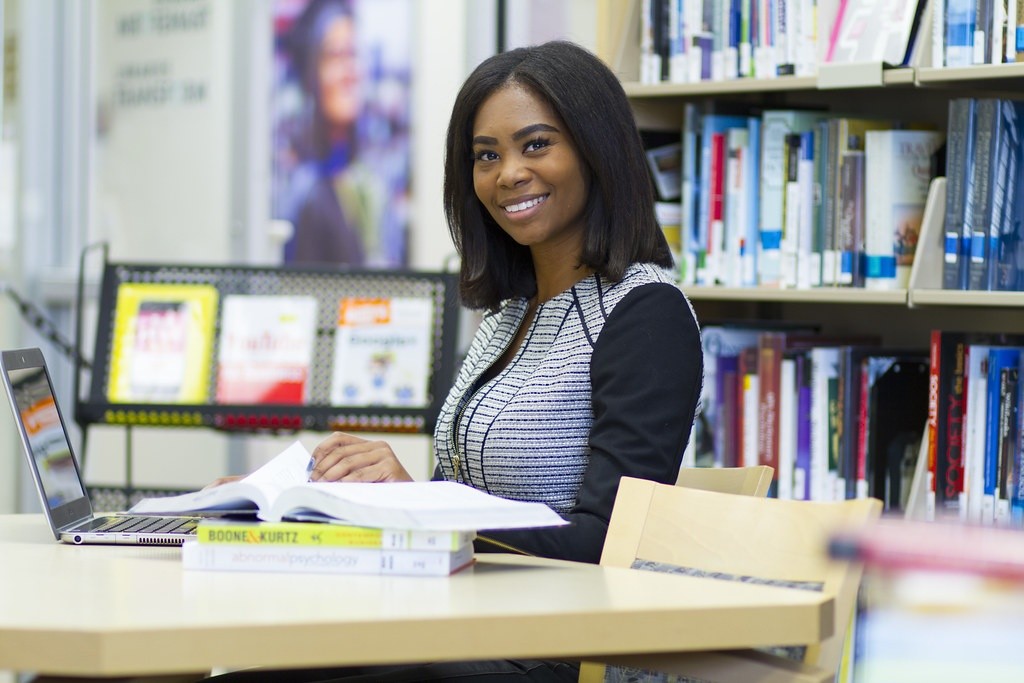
[0,507,838,683]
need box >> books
[636,98,1024,294]
[126,439,570,531]
[183,520,477,576]
[595,0,1024,85]
[684,323,1024,533]
[218,292,314,406]
[104,282,216,406]
[329,295,434,410]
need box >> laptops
[0,348,330,546]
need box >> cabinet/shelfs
[572,0,1023,340]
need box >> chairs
[575,463,880,683]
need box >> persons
[269,1,412,275]
[305,40,704,565]
